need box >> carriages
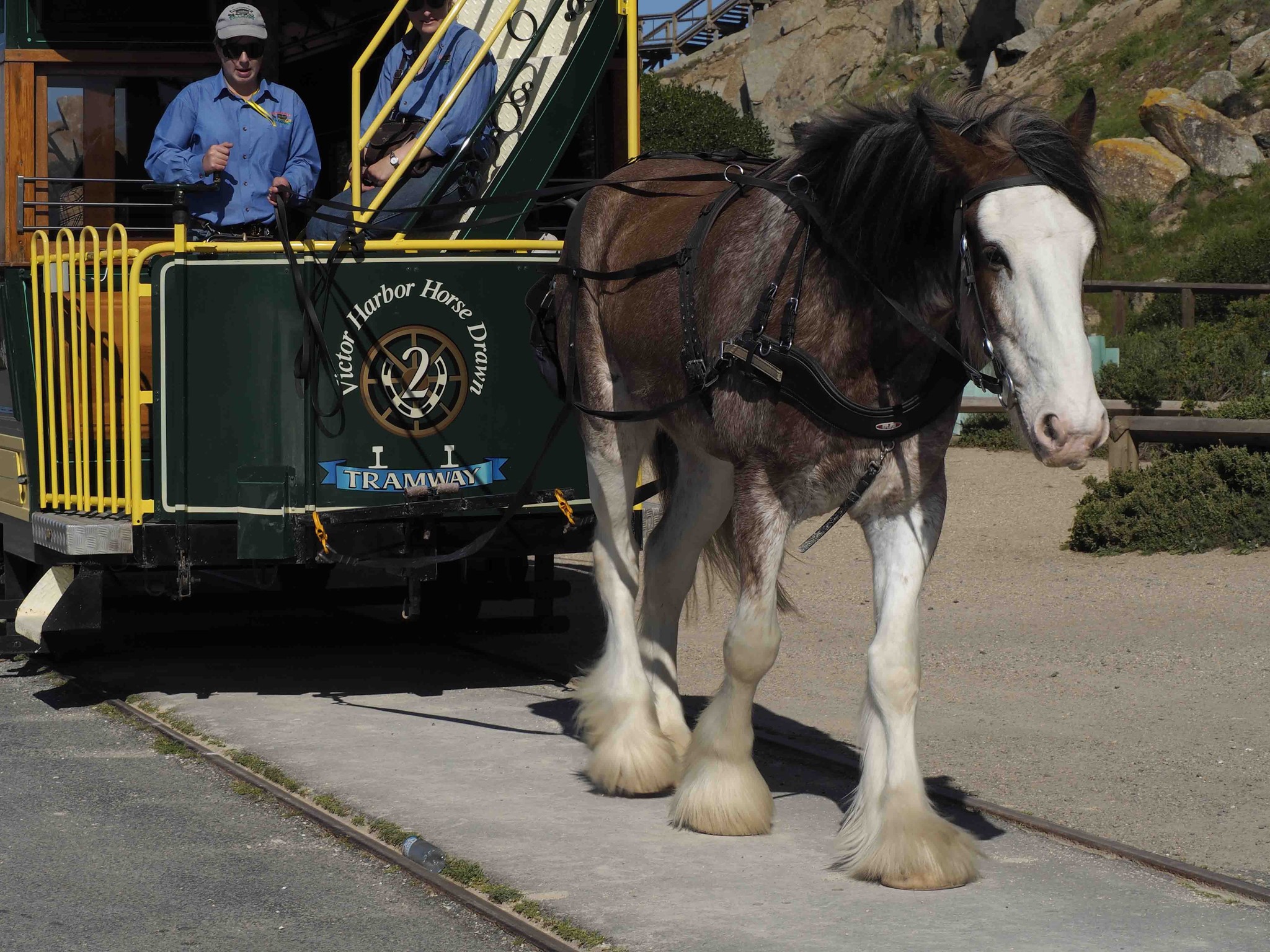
[0,0,1133,911]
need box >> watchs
[390,150,400,169]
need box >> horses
[556,85,1112,891]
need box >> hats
[215,2,267,40]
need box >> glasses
[219,42,266,59]
[405,1,448,12]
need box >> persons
[145,0,323,242]
[304,0,500,242]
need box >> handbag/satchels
[364,118,435,178]
